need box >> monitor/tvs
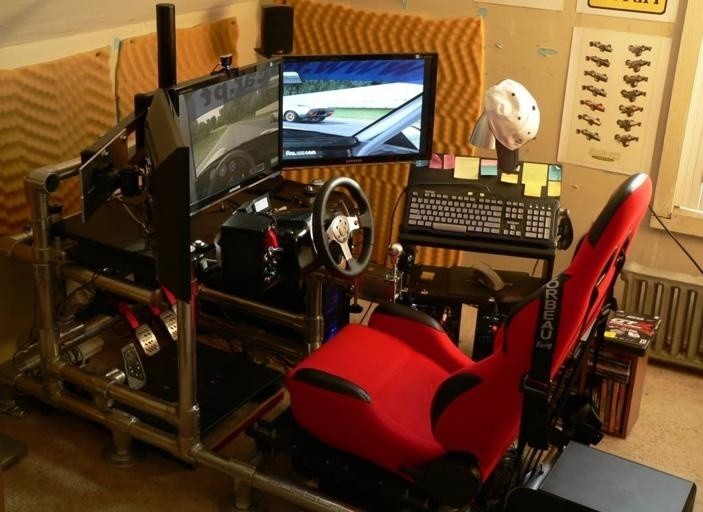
[281,53,439,168]
[143,88,192,304]
[169,56,282,219]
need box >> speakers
[261,3,294,55]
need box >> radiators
[618,261,703,372]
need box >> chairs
[286,172,652,507]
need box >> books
[548,309,662,435]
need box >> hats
[485,79,540,150]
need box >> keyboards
[399,151,563,250]
